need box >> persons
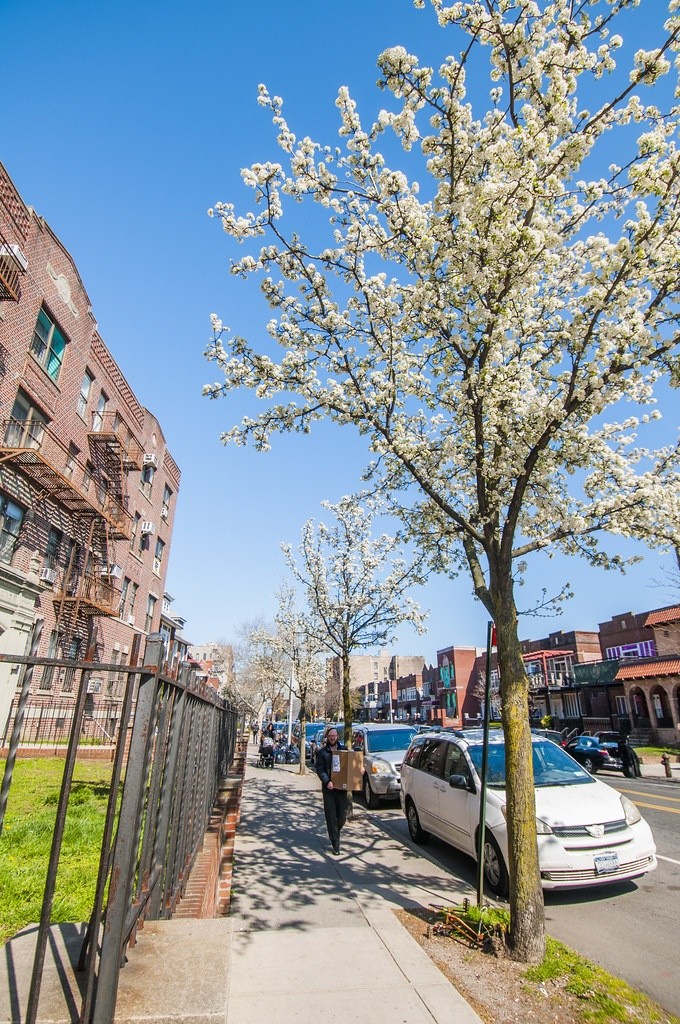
[260,723,275,765]
[252,721,259,744]
[316,728,365,855]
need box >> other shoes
[332,848,340,855]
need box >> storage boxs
[330,750,364,791]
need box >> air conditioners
[162,508,168,518]
[40,568,58,584]
[86,681,96,694]
[144,453,158,466]
[102,565,123,579]
[0,244,29,271]
[142,522,156,535]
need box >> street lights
[287,630,307,746]
[373,661,393,724]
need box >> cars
[262,718,360,769]
[352,722,420,809]
[564,730,636,777]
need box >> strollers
[257,734,275,769]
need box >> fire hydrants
[661,752,672,777]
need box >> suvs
[399,726,658,898]
